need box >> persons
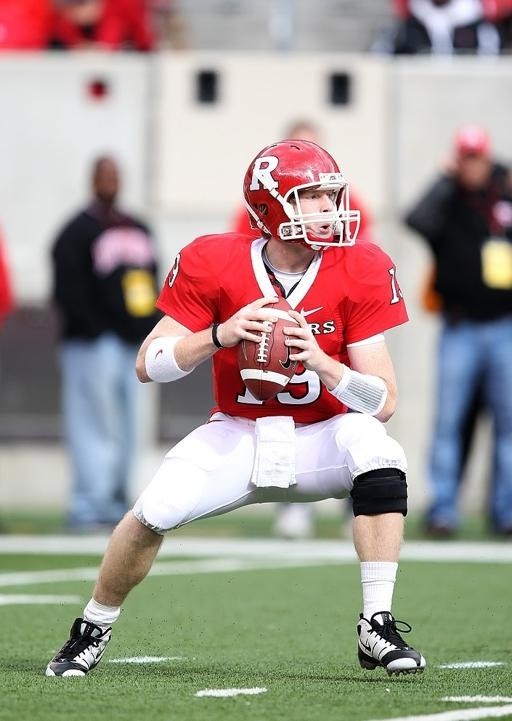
[49,158,158,527]
[399,120,511,547]
[0,2,159,53]
[365,0,512,56]
[46,137,427,682]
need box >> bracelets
[212,323,224,350]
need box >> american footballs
[238,295,299,401]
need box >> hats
[457,126,488,157]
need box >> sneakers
[45,615,111,679]
[356,611,426,677]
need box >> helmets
[242,138,360,247]
